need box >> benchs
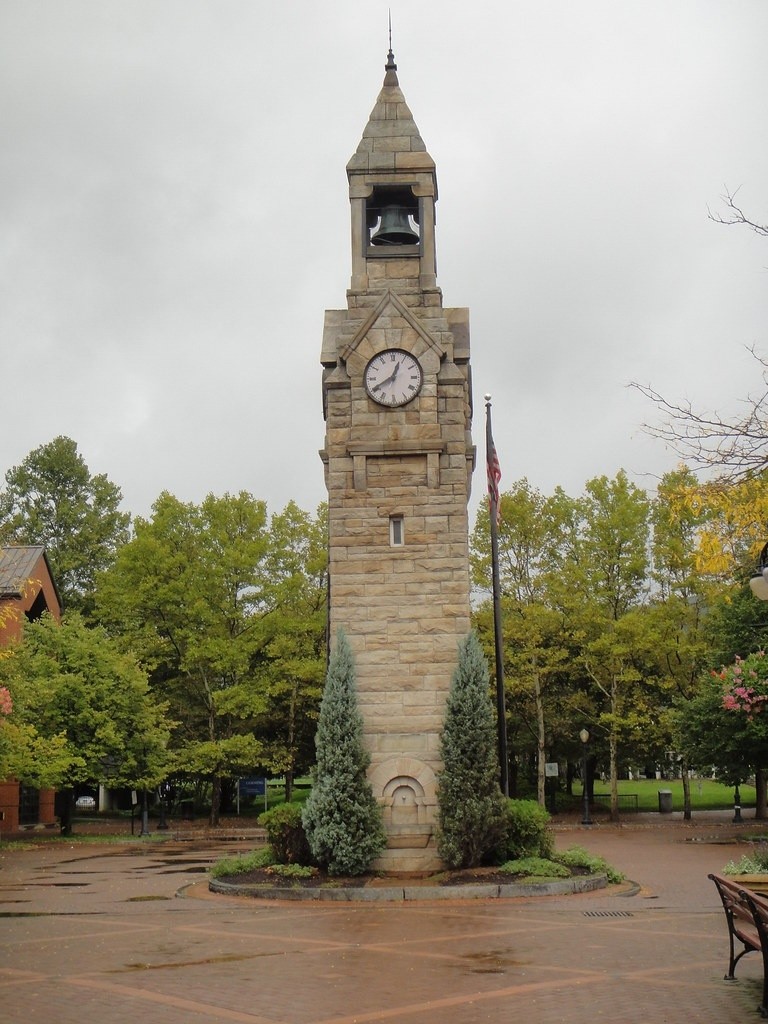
[707,873,768,1019]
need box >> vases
[723,874,768,918]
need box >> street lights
[579,728,590,823]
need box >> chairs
[76,796,95,811]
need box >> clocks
[362,348,425,408]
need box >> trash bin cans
[181,800,194,820]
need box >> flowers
[721,855,768,874]
